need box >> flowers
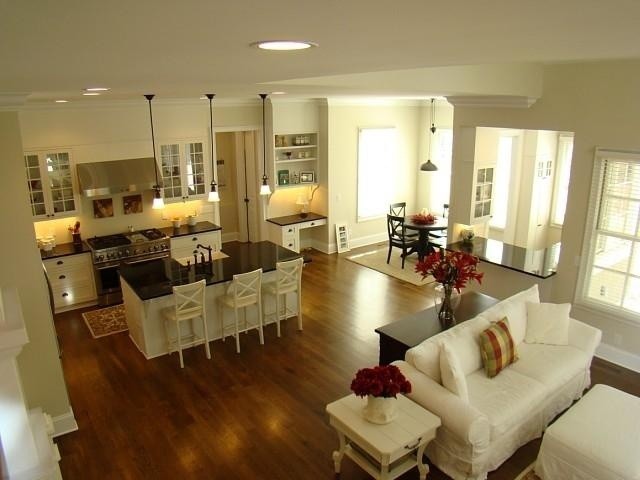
[413,247,484,295]
[349,364,412,398]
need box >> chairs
[217,268,265,353]
[429,204,450,237]
[262,258,304,337]
[386,214,416,269]
[428,237,447,258]
[159,279,212,368]
[389,202,420,238]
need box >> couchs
[389,285,602,480]
[534,384,640,479]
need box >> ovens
[91,252,167,296]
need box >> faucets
[199,252,205,264]
[196,244,212,264]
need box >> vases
[362,394,399,425]
[433,284,462,321]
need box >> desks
[374,291,501,366]
[400,215,448,264]
[118,241,303,360]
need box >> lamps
[421,98,439,171]
[204,93,221,201]
[258,94,272,195]
[144,94,166,210]
[297,194,309,218]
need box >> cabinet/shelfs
[449,128,497,225]
[266,211,327,254]
[264,97,321,191]
[158,221,222,258]
[41,240,99,315]
[24,147,80,223]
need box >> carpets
[516,461,545,480]
[346,241,437,287]
[81,303,129,339]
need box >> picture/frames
[299,171,315,184]
[335,223,350,254]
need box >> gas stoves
[84,226,171,265]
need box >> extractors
[76,157,164,200]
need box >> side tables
[325,387,441,478]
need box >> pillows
[438,341,470,401]
[525,300,571,345]
[480,316,520,378]
